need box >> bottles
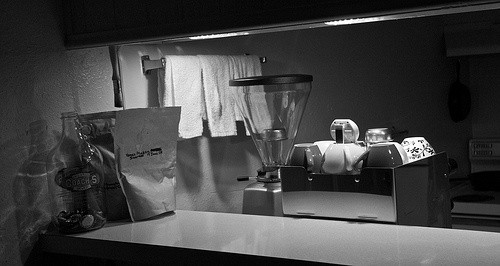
[46,112,107,234]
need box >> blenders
[229,73,313,216]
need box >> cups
[401,137,436,165]
[289,143,322,172]
[354,144,403,168]
[367,128,389,148]
[321,143,365,174]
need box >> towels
[196,54,272,138]
[157,53,203,142]
